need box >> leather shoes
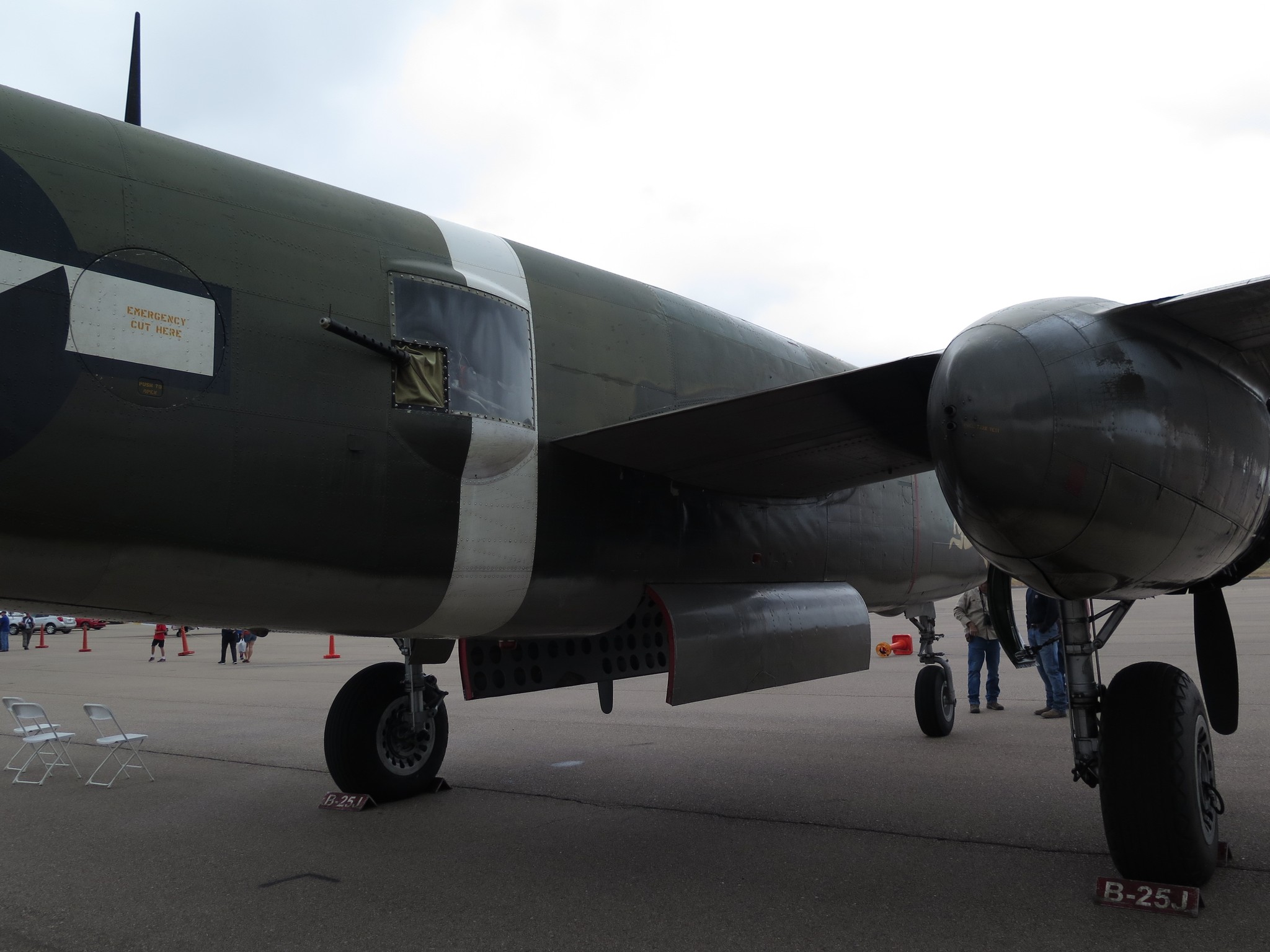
[1033,706,1052,715]
[1040,708,1067,718]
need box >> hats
[0,611,6,613]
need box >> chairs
[83,703,155,788]
[2,697,71,773]
[10,703,81,786]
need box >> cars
[75,618,106,630]
[9,613,77,635]
[0,611,26,621]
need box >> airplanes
[1,14,1269,901]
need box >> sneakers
[157,658,166,663]
[218,660,226,665]
[970,704,980,713]
[148,657,155,662]
[986,702,1004,711]
[232,661,238,664]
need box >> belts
[1028,623,1041,628]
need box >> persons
[217,628,238,665]
[1025,587,1069,719]
[18,612,36,650]
[234,630,246,659]
[0,611,10,652]
[148,624,167,663]
[240,629,257,662]
[953,580,1005,714]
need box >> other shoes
[0,650,6,652]
[26,647,29,650]
[242,658,249,662]
[247,660,250,663]
[24,647,27,650]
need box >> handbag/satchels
[19,624,26,630]
[238,638,247,653]
